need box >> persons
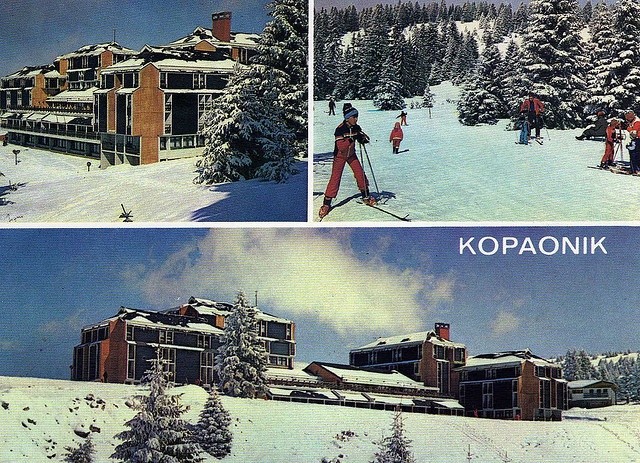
[601,119,620,168]
[575,111,608,140]
[397,111,408,126]
[518,116,531,145]
[623,110,640,176]
[519,94,545,140]
[329,99,336,115]
[390,122,403,154]
[318,102,369,217]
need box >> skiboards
[312,198,410,223]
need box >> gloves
[390,140,391,142]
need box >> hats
[598,112,604,116]
[343,103,358,119]
[611,120,617,126]
[625,112,633,119]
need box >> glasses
[529,98,532,99]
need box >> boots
[575,134,585,140]
[395,148,398,154]
[586,135,590,140]
[393,146,395,154]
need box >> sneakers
[329,113,331,115]
[601,162,608,169]
[519,140,524,144]
[524,141,528,145]
[361,189,376,205]
[527,135,531,139]
[536,134,540,140]
[608,160,616,166]
[318,198,332,218]
[406,124,407,126]
[333,113,335,115]
[401,124,402,125]
